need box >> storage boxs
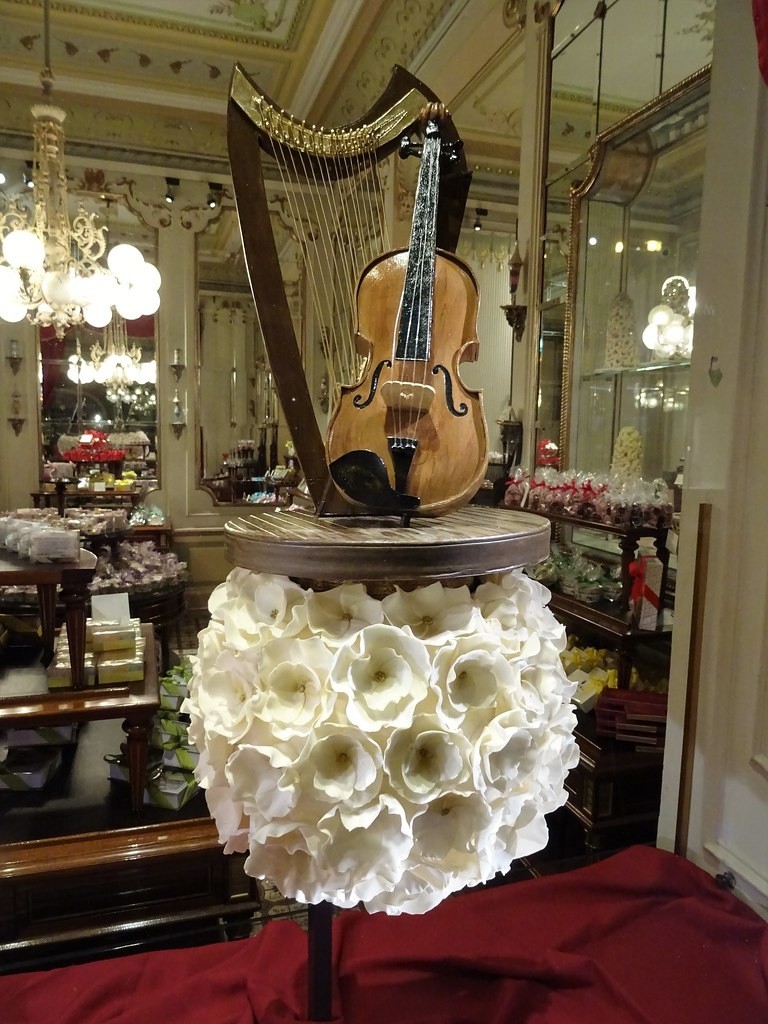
[566,670,603,714]
[560,649,576,676]
[571,646,593,671]
[588,666,609,686]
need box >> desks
[76,513,170,579]
[0,540,96,690]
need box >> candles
[174,348,180,366]
[10,341,20,358]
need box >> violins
[326,99,490,514]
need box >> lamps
[604,198,640,370]
[106,381,132,405]
[23,160,39,189]
[207,194,217,209]
[472,218,483,232]
[130,384,156,412]
[165,177,182,202]
[0,0,163,343]
[66,306,141,385]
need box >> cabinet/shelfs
[498,500,675,689]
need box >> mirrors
[40,220,160,507]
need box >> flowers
[181,565,577,913]
